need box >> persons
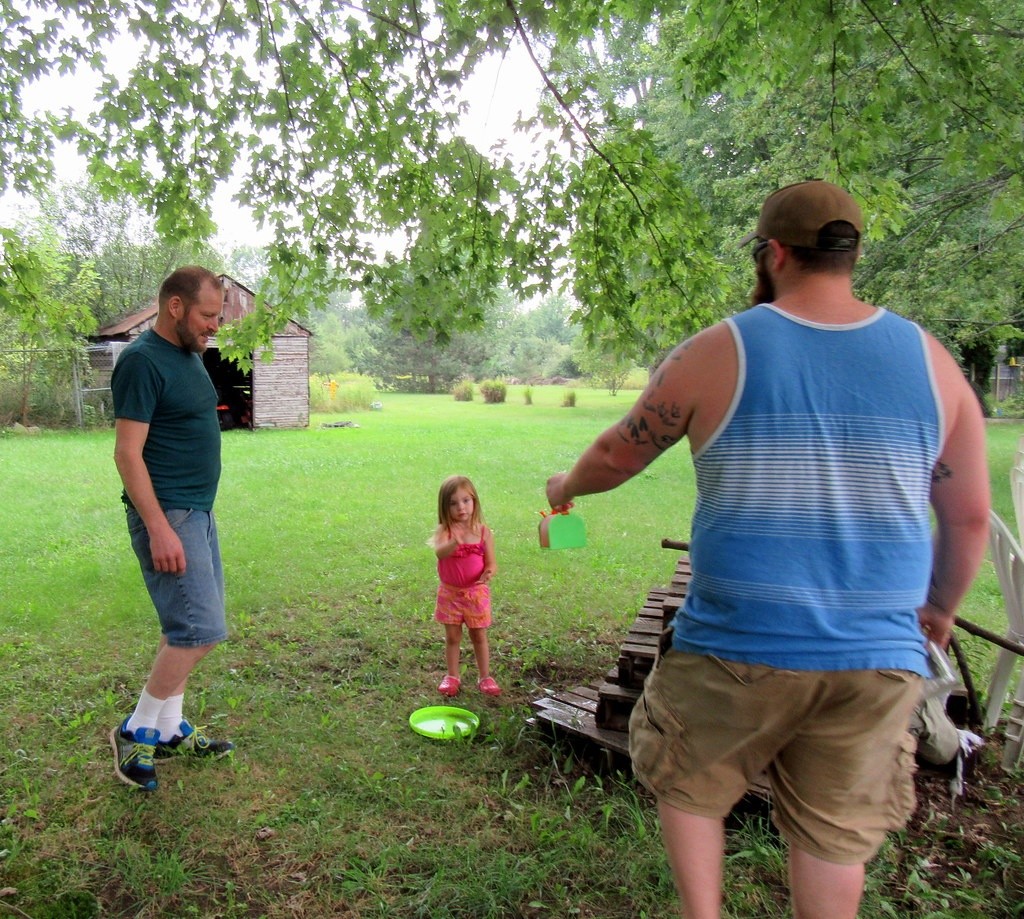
[544,182,988,919]
[108,265,236,790]
[432,475,500,696]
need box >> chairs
[977,435,1024,769]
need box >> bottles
[920,625,959,697]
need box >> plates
[409,706,480,740]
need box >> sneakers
[152,722,232,764]
[110,717,159,790]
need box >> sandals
[439,674,461,696]
[477,675,499,696]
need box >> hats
[736,177,864,254]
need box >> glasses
[752,240,783,266]
